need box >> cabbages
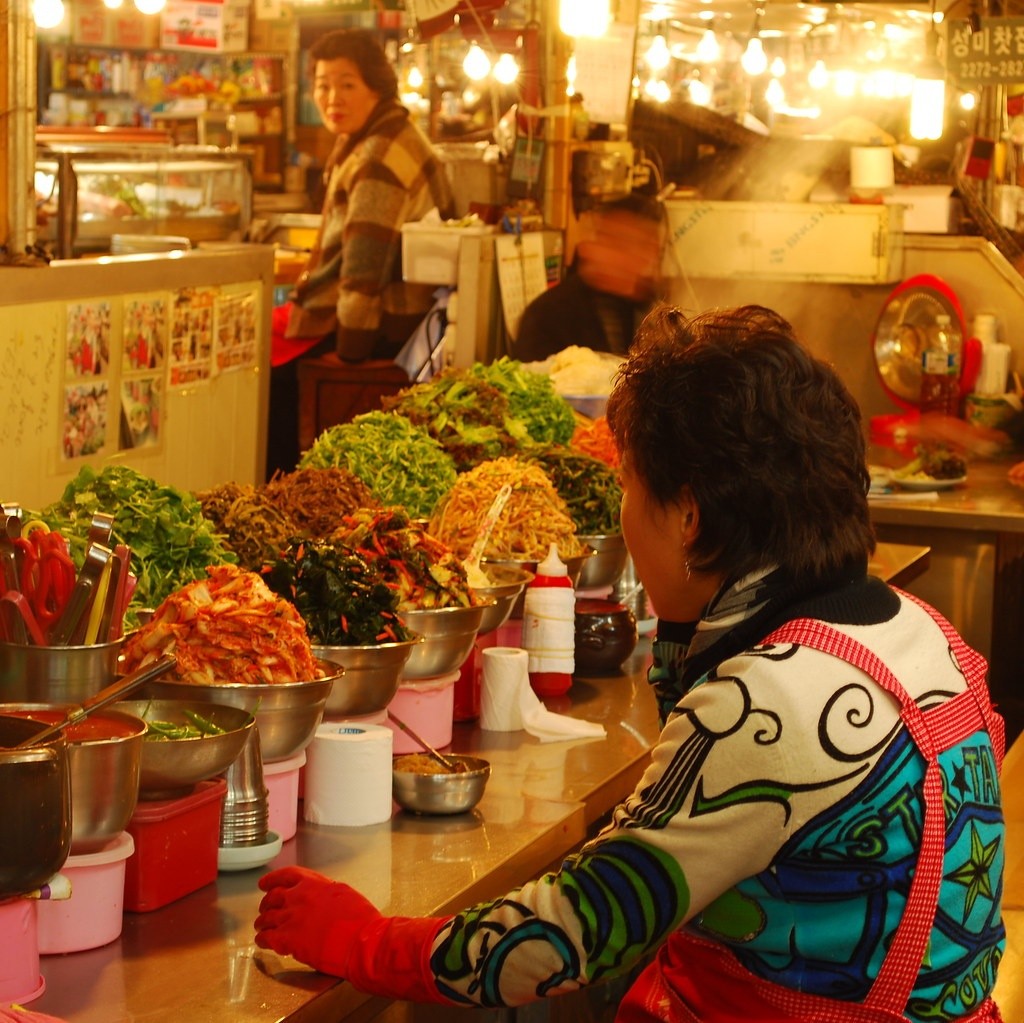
[112,566,328,686]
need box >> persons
[267,30,460,481]
[512,188,664,361]
[268,313,999,1022]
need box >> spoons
[459,482,511,588]
[387,709,470,773]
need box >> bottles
[990,132,1019,229]
[521,542,572,695]
[920,313,958,418]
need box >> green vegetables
[447,355,576,453]
[19,465,236,642]
[295,408,458,519]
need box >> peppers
[135,694,262,740]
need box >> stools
[297,355,411,468]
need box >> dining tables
[868,457,1024,752]
[20,543,932,1023]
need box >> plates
[218,828,283,871]
[889,477,967,490]
[110,233,190,253]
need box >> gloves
[252,864,474,1011]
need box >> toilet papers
[481,646,528,734]
[299,721,394,826]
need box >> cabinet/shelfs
[34,35,288,201]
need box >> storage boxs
[67,2,161,47]
[159,0,249,53]
[399,222,494,286]
[881,186,962,233]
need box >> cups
[970,314,1009,397]
[0,639,127,703]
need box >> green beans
[550,457,622,536]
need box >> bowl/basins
[0,704,149,898]
[398,593,490,681]
[560,389,617,422]
[220,724,269,849]
[464,531,629,632]
[117,654,347,764]
[392,754,489,813]
[573,601,639,678]
[307,629,423,715]
[850,145,898,190]
[105,702,255,787]
[966,395,1022,431]
